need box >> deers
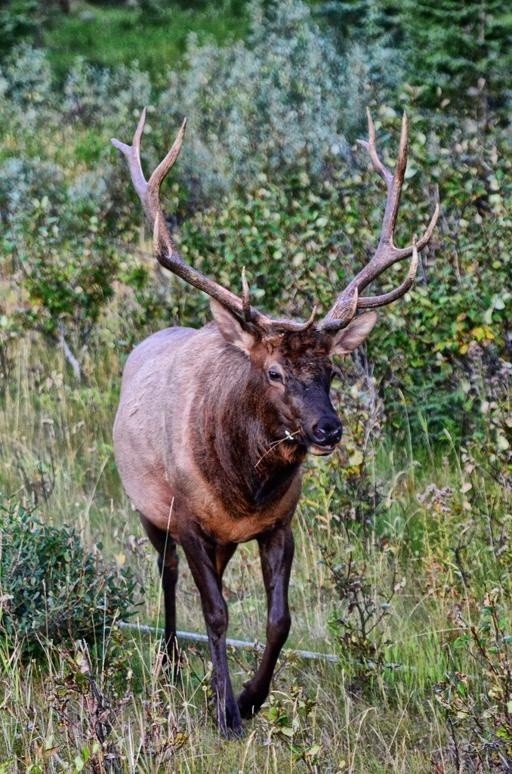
[110,104,441,739]
